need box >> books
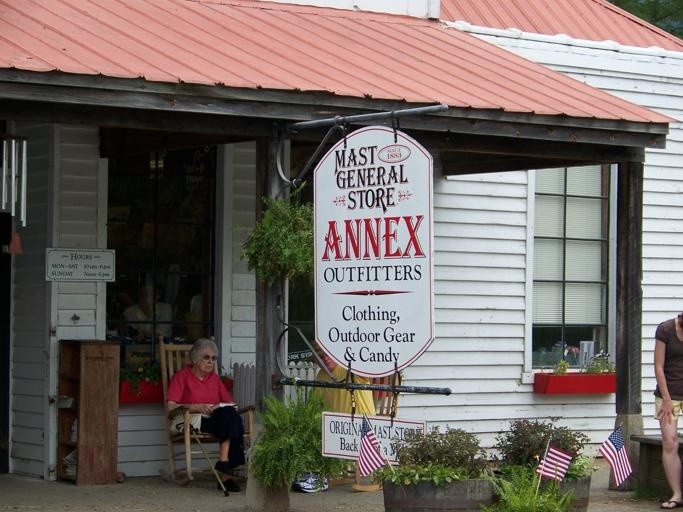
[211,402,236,410]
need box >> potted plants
[373,415,594,510]
[246,384,354,494]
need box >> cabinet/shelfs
[56,339,122,486]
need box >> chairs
[159,336,255,486]
[110,288,209,331]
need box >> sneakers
[216,480,244,496]
[213,461,245,482]
[291,472,329,493]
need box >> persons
[291,340,375,496]
[115,278,173,342]
[652,312,682,509]
[167,336,244,491]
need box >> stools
[630,431,683,501]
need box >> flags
[599,426,633,487]
[535,443,575,484]
[358,416,387,481]
